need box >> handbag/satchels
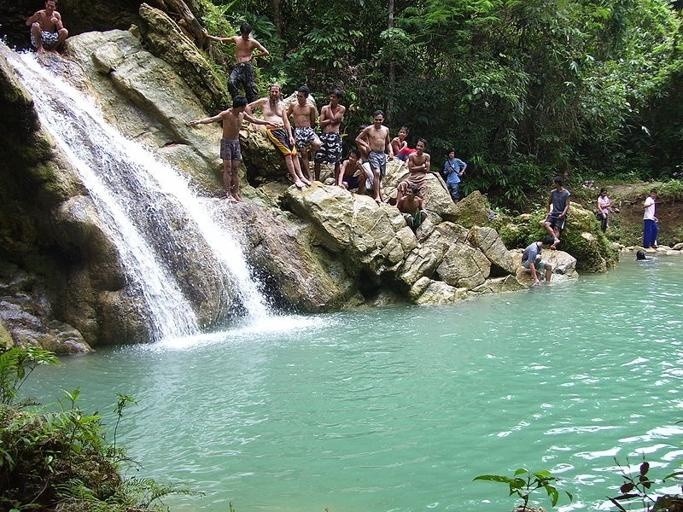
[460,167,467,179]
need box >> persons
[642,188,660,249]
[521,234,553,285]
[184,96,279,203]
[242,82,468,228]
[543,176,572,251]
[26,1,68,56]
[595,187,613,234]
[635,250,648,261]
[202,23,271,112]
